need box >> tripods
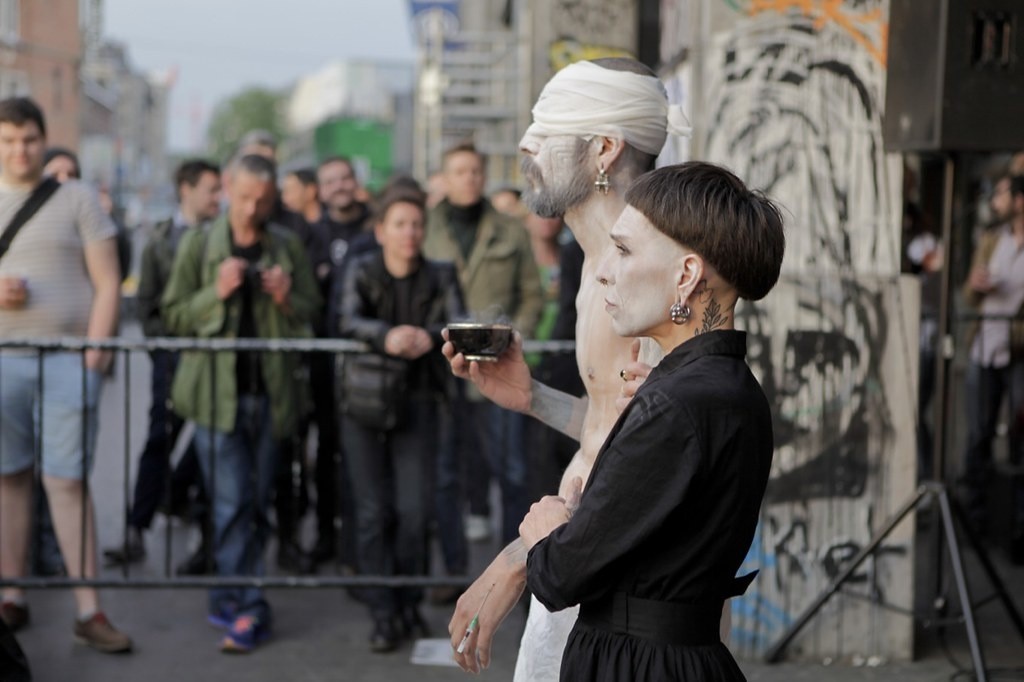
[766,158,1024,682]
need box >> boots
[394,587,430,639]
[340,584,399,652]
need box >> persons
[43,124,586,655]
[441,56,662,682]
[1,95,134,654]
[903,151,1024,456]
[520,162,785,682]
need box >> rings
[619,369,627,381]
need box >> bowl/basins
[449,324,512,361]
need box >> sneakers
[206,588,251,629]
[0,603,29,633]
[218,602,270,652]
[72,612,131,653]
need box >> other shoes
[176,549,218,575]
[430,584,462,605]
[277,541,320,576]
[310,537,336,560]
[104,544,145,563]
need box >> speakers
[883,1,1023,154]
